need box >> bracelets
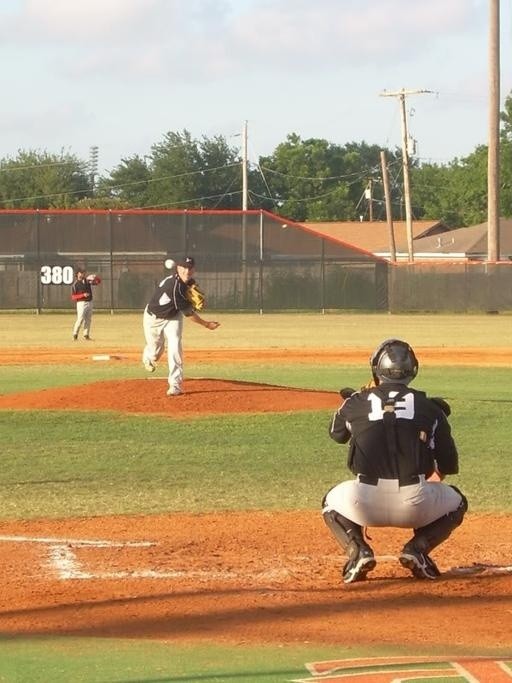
[204,321,211,327]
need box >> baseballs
[164,259,175,268]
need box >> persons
[139,255,220,396]
[70,266,95,340]
[319,339,468,584]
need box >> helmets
[370,338,418,385]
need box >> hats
[74,267,85,274]
[177,256,195,268]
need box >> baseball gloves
[185,283,205,312]
[86,274,100,285]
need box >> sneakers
[142,358,155,372]
[84,335,89,339]
[73,335,77,339]
[343,548,376,583]
[167,385,180,395]
[399,545,442,579]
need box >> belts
[359,474,420,487]
